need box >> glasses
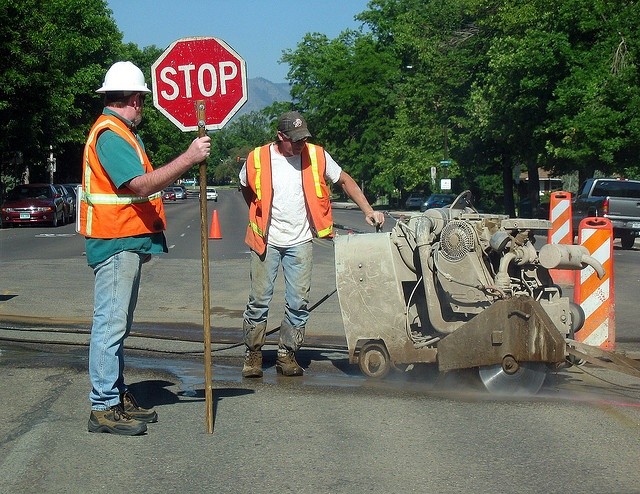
[285,138,308,144]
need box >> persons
[240,113,385,377]
[75,62,211,435]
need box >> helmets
[95,60,152,94]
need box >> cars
[406,193,427,210]
[175,186,187,200]
[2,184,77,227]
[420,191,451,212]
[163,188,177,203]
[198,187,218,202]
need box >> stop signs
[149,37,251,133]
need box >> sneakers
[86,407,147,436]
[276,352,304,376]
[241,348,264,378]
[118,391,157,423]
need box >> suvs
[538,173,640,255]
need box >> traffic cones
[575,213,619,357]
[208,208,223,239]
[546,185,574,290]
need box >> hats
[278,111,312,141]
